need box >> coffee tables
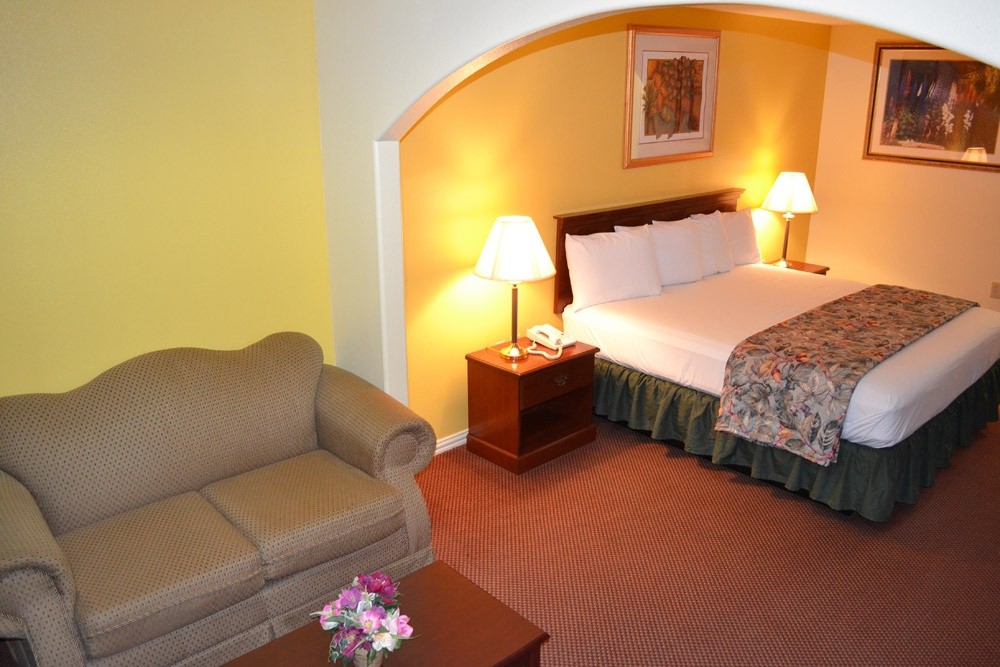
[221,559,551,667]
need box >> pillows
[561,227,661,313]
[690,207,761,267]
[650,211,734,286]
[608,221,703,287]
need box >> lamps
[473,216,556,360]
[761,170,817,267]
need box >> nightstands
[767,259,829,275]
[464,333,601,475]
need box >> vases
[352,642,384,667]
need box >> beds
[552,187,1000,525]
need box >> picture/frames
[623,22,721,169]
[861,39,999,174]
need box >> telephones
[526,323,576,351]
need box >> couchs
[0,332,436,667]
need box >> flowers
[309,570,422,664]
